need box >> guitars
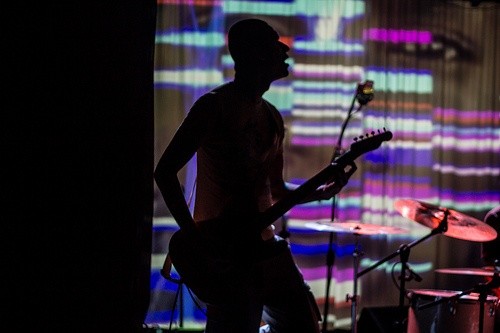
[167,129,394,302]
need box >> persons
[154,18,358,333]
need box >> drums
[434,263,500,293]
[407,285,498,333]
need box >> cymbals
[304,220,406,236]
[393,199,497,242]
